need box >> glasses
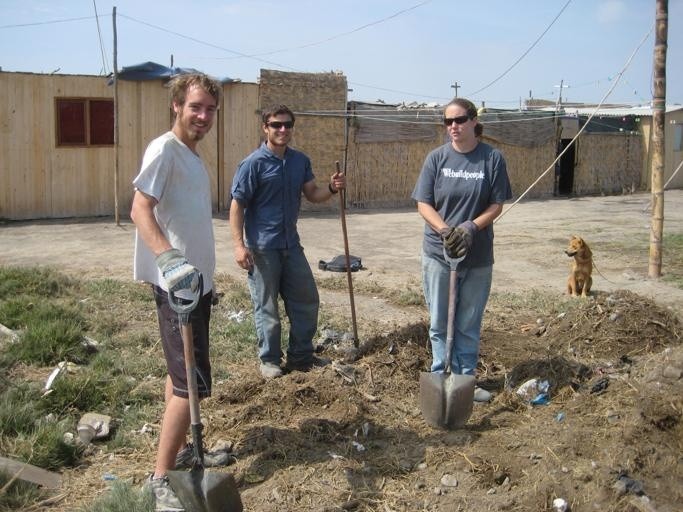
[443,115,473,126]
[266,121,293,129]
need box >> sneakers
[259,360,283,378]
[141,473,186,512]
[473,383,493,402]
[175,442,230,468]
[285,351,333,372]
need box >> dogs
[564,233,594,298]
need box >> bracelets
[328,184,335,195]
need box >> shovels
[419,247,476,430]
[164,269,243,512]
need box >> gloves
[154,247,200,294]
[445,219,480,258]
[439,227,457,258]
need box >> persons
[411,97,513,403]
[229,105,347,378]
[131,73,229,512]
